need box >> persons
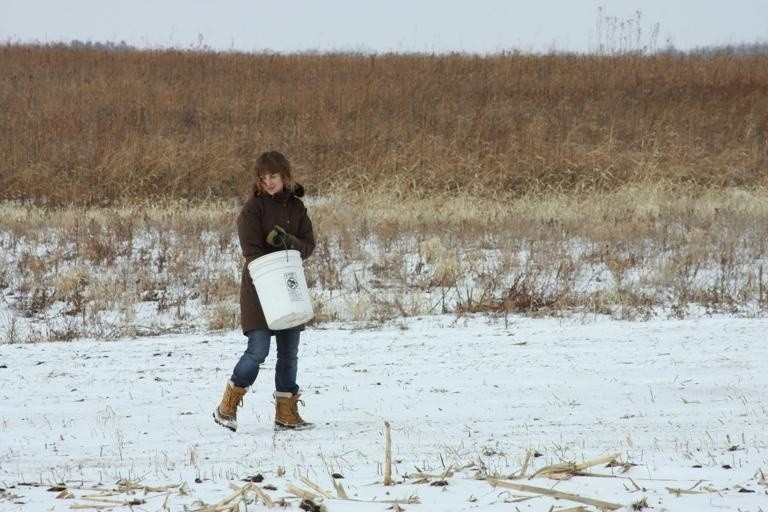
[212,151,314,432]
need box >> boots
[211,378,250,433]
[271,389,314,432]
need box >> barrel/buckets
[247,250,314,330]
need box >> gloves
[265,225,288,248]
